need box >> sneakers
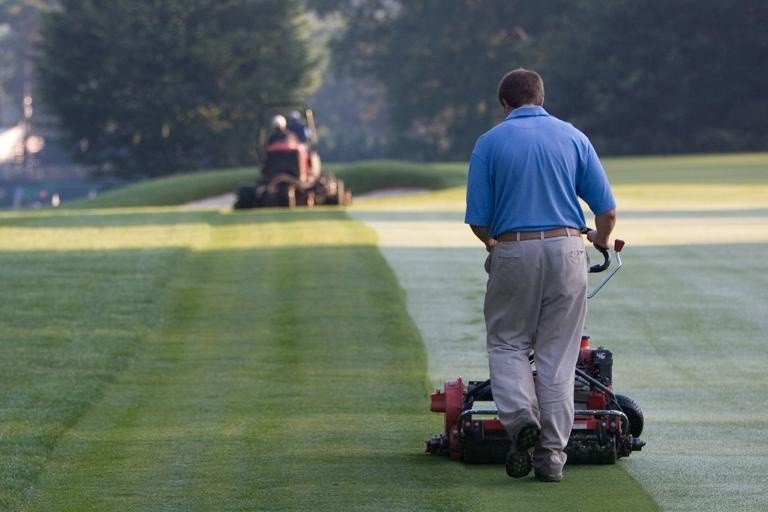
[534,467,559,482]
[505,415,541,479]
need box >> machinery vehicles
[237,102,353,207]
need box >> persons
[287,110,312,145]
[461,66,617,483]
[267,114,292,145]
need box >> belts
[496,229,579,240]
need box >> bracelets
[485,246,494,252]
